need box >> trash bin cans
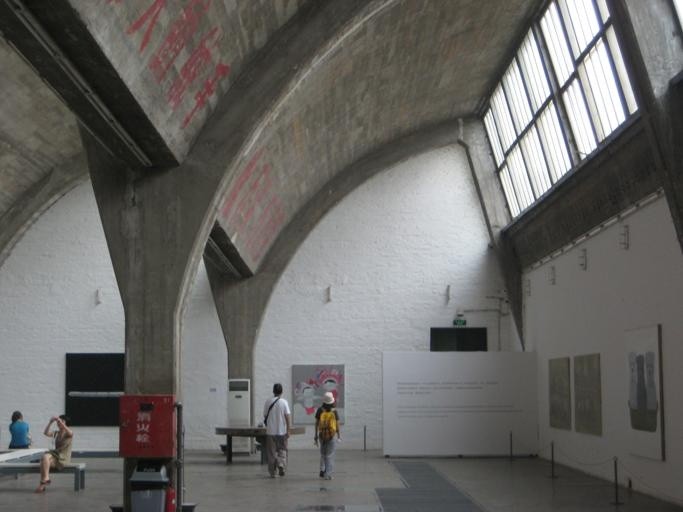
[128,463,170,512]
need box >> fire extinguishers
[166,483,176,512]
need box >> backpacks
[318,409,338,440]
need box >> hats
[322,391,336,405]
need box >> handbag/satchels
[264,417,268,426]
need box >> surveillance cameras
[504,295,509,303]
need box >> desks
[216,423,308,466]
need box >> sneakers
[319,470,335,480]
[278,466,285,476]
[41,479,51,485]
[35,488,45,494]
[270,472,277,478]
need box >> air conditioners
[226,378,251,455]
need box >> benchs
[0,446,52,462]
[69,447,120,459]
[0,461,85,491]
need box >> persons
[8,411,33,480]
[314,392,341,480]
[263,383,292,478]
[35,415,73,493]
